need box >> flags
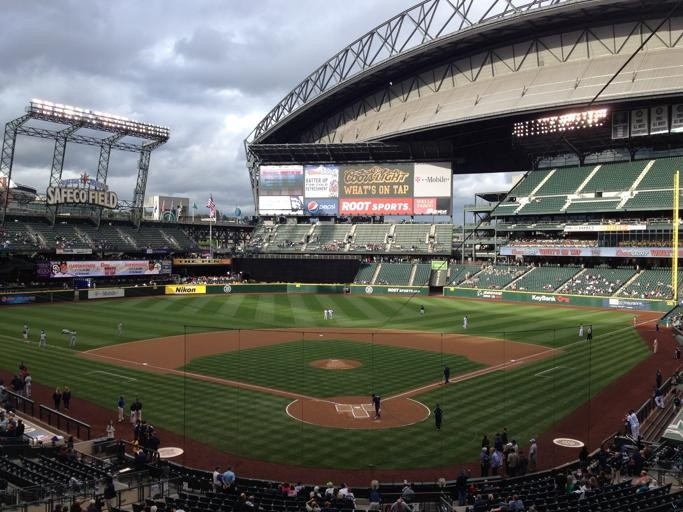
[149,193,216,217]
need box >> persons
[0,216,683,512]
